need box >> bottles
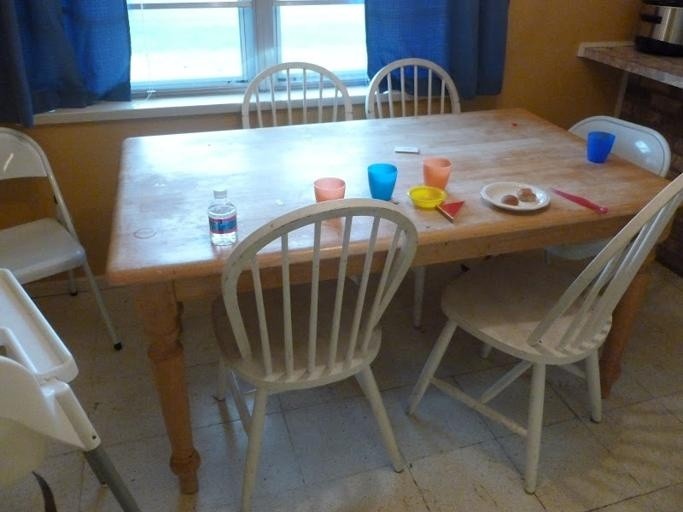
[207,187,237,248]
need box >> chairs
[364,57,461,330]
[239,61,352,129]
[408,171,683,493]
[208,197,419,512]
[478,115,672,358]
[0,267,139,512]
[0,126,123,351]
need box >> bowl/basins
[407,186,447,209]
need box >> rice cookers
[633,0,683,58]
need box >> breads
[501,195,518,205]
[517,188,536,202]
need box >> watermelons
[436,201,464,222]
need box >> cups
[586,131,615,164]
[312,177,346,203]
[367,163,397,201]
[422,157,452,191]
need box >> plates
[480,182,551,211]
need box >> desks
[577,39,683,278]
[105,107,672,493]
